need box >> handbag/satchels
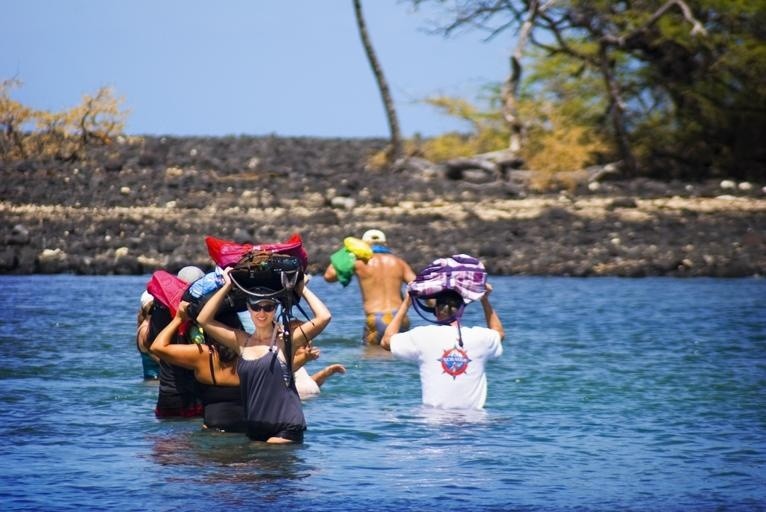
[329,233,374,288]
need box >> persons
[196,267,331,445]
[139,266,346,434]
[380,280,507,410]
[325,230,417,346]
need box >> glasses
[247,302,280,314]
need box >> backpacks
[201,231,309,311]
[146,265,247,346]
[406,253,489,324]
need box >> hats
[245,286,278,305]
[363,227,387,245]
[140,290,155,310]
